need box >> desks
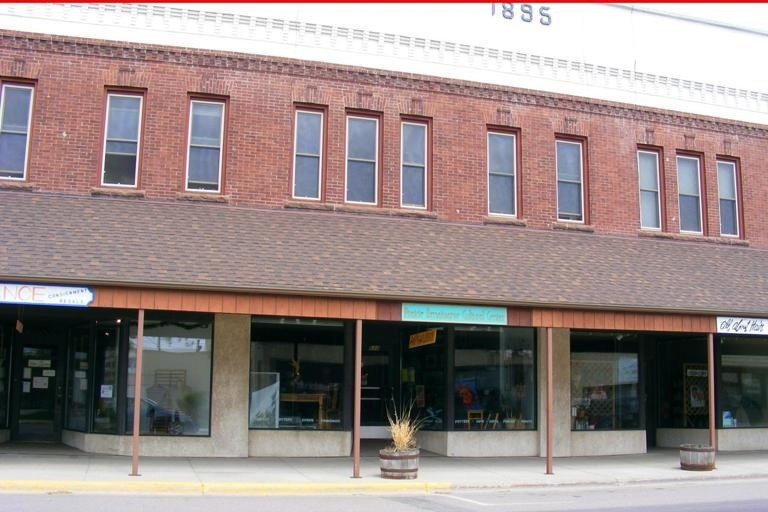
[279,393,325,431]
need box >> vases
[679,444,716,471]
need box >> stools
[467,409,484,431]
[484,412,500,430]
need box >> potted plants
[378,392,435,480]
